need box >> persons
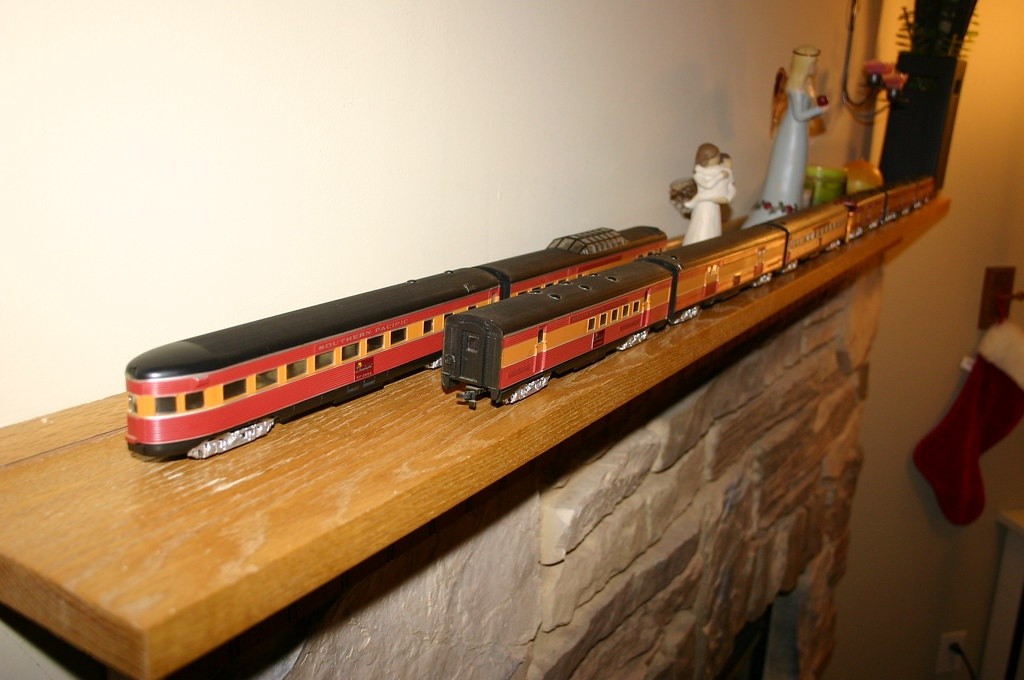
[669,143,737,247]
[741,45,829,229]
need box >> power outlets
[935,630,967,674]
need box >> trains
[443,168,938,410]
[125,225,666,461]
